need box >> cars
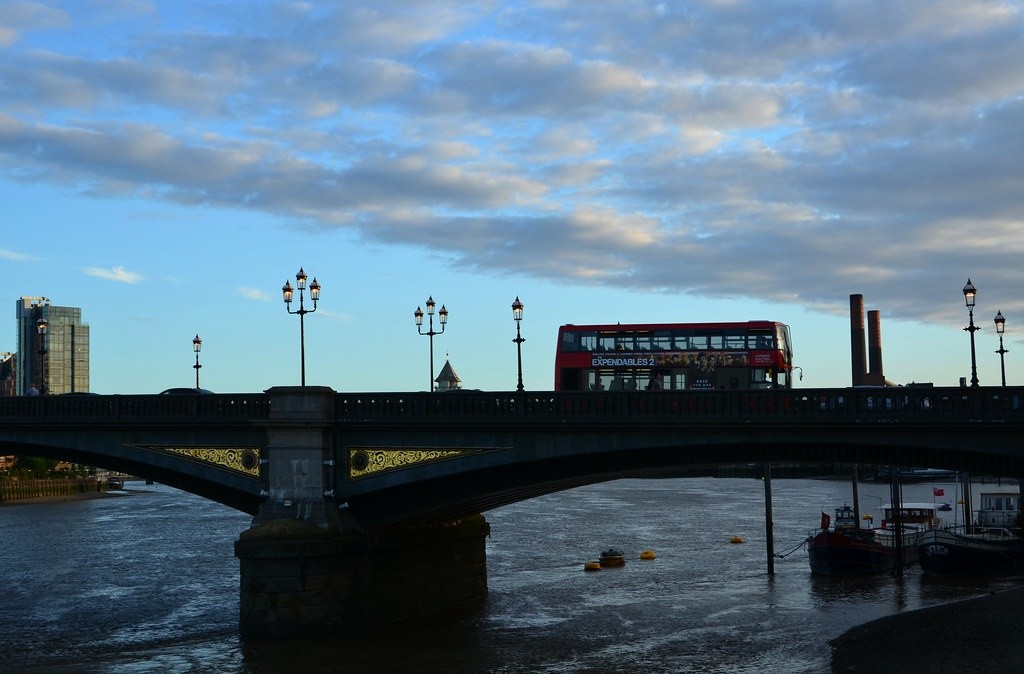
[160,386,215,395]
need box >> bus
[554,320,803,392]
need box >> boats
[598,545,625,567]
[915,485,1024,579]
[867,503,941,553]
[803,510,876,578]
[832,503,857,529]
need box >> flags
[821,512,830,531]
[934,488,944,496]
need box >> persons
[756,336,772,348]
[648,352,748,390]
[648,372,662,390]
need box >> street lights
[281,267,321,385]
[36,310,49,395]
[962,278,981,387]
[191,333,203,388]
[993,309,1009,386]
[510,295,527,392]
[413,295,449,392]
